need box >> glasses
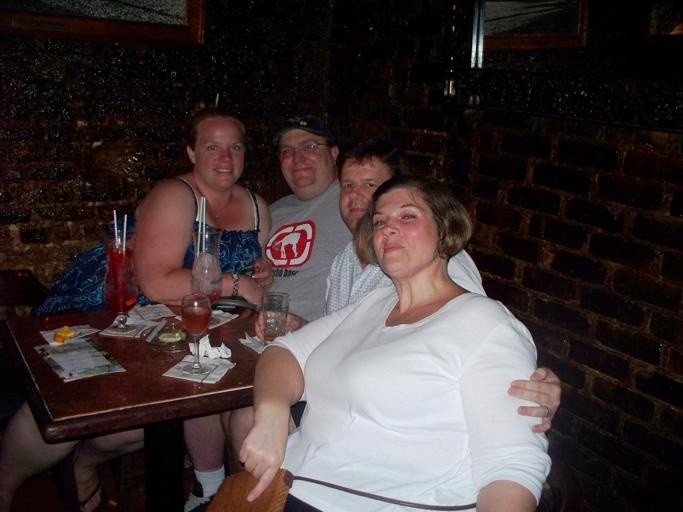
[276,143,328,159]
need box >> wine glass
[192,225,223,324]
[182,291,213,375]
[105,229,137,334]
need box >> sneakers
[184,480,215,512]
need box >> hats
[265,115,332,144]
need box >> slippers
[76,477,105,512]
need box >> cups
[160,321,187,355]
[263,291,289,347]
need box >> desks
[0,296,261,512]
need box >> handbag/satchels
[207,468,293,512]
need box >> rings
[545,406,549,416]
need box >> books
[32,336,127,383]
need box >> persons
[182,112,353,512]
[228,139,562,454]
[0,105,273,512]
[235,171,552,512]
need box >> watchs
[231,273,238,296]
